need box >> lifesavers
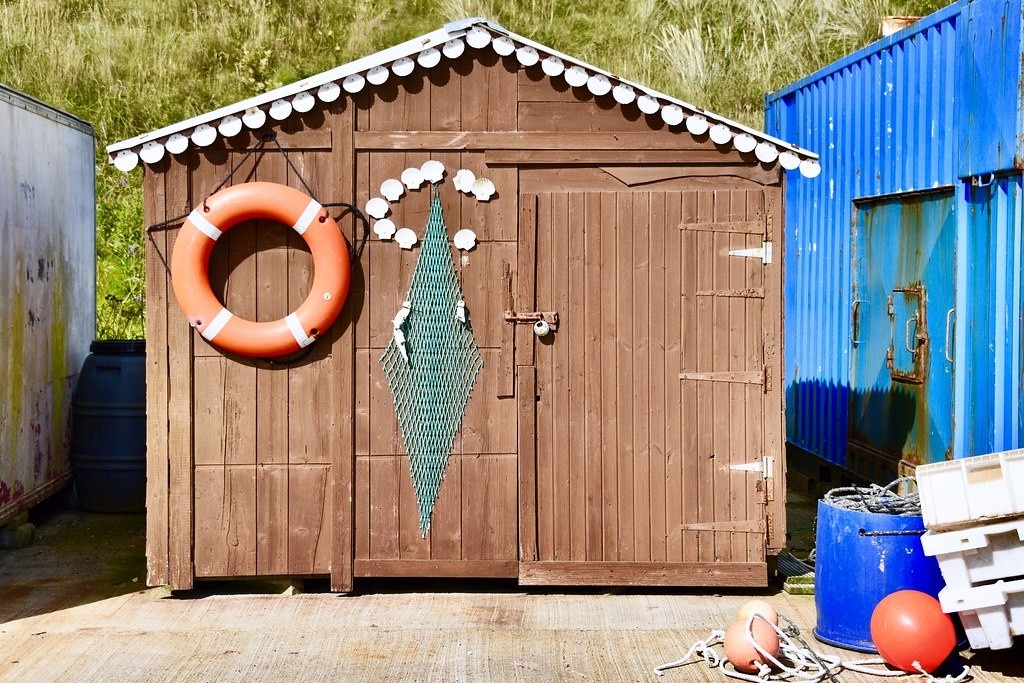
[171,181,351,359]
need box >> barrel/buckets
[71,337,149,512]
[812,499,968,654]
